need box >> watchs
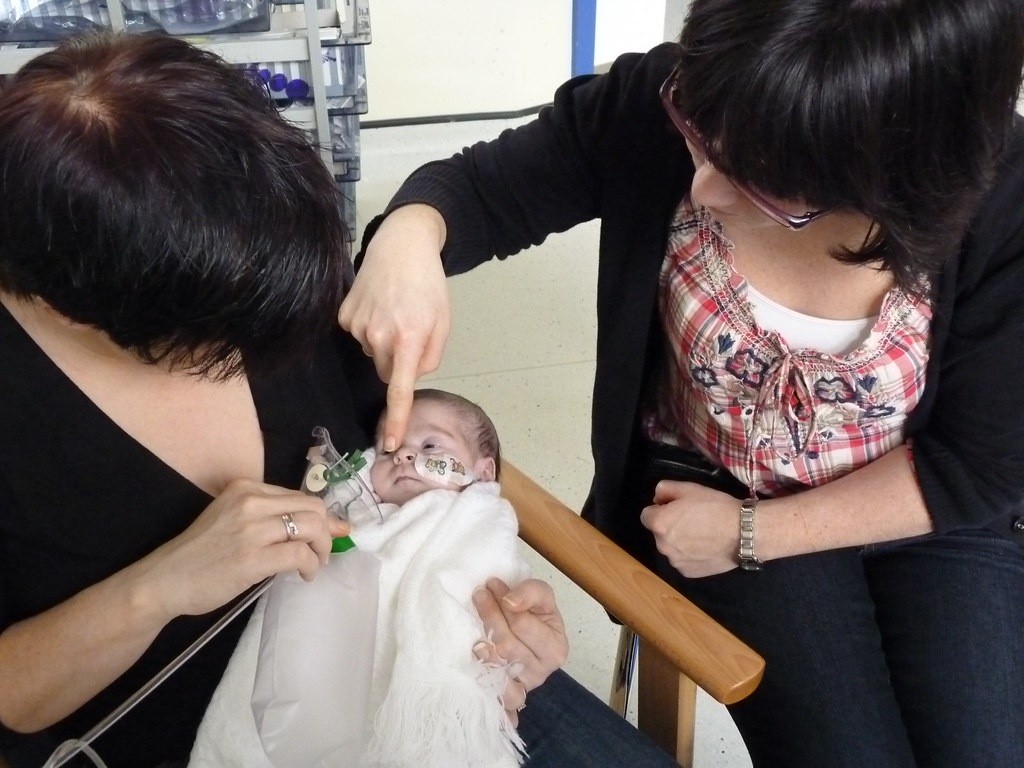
[738,498,762,571]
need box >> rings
[281,513,299,541]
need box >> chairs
[496,457,770,768]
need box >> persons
[339,1,1024,768]
[0,30,685,768]
[185,388,527,767]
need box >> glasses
[658,59,845,232]
